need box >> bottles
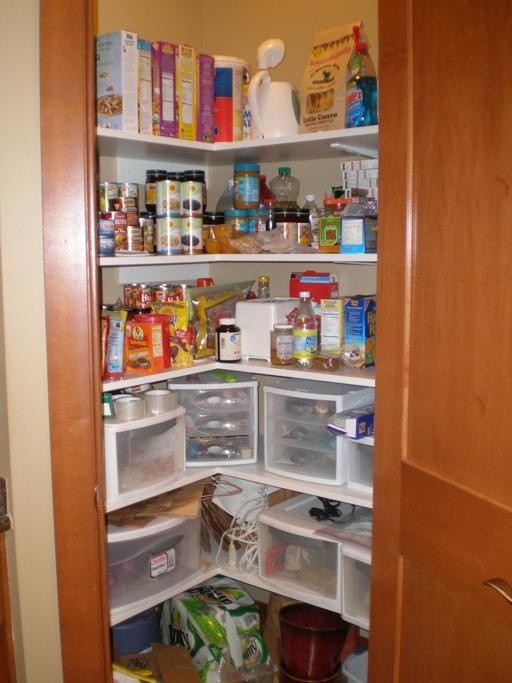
[184,168,208,212]
[293,292,318,359]
[145,169,166,212]
[248,210,266,235]
[137,313,170,370]
[268,167,300,210]
[215,179,233,212]
[265,210,272,232]
[233,162,259,209]
[214,317,242,363]
[259,174,276,207]
[296,208,311,246]
[224,210,247,235]
[269,323,294,366]
[272,208,298,244]
[303,194,319,242]
[256,277,270,299]
[319,198,342,253]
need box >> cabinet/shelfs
[93,125,380,682]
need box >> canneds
[155,179,180,216]
[98,181,153,256]
[155,216,180,256]
[181,180,202,217]
[181,217,202,255]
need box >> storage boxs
[106,501,202,614]
[341,541,371,630]
[104,404,186,496]
[346,431,374,496]
[259,495,362,605]
[169,372,258,469]
[264,378,371,485]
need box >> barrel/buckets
[277,602,352,682]
